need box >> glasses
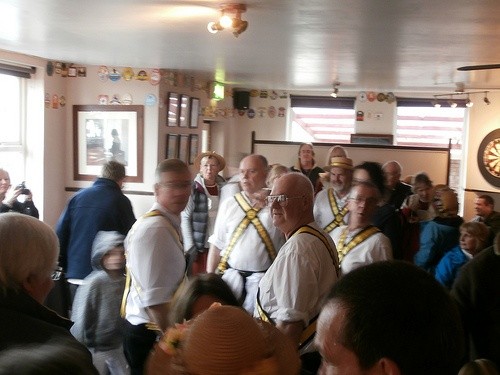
[52,266,62,280]
[264,195,303,207]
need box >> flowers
[158,301,281,375]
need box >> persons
[0,168,39,219]
[0,212,102,375]
[398,173,500,357]
[55,161,138,318]
[69,230,133,375]
[146,302,302,375]
[183,143,412,301]
[252,171,339,375]
[119,158,193,375]
[158,272,243,327]
[312,260,472,374]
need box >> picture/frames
[72,104,144,184]
[352,133,394,145]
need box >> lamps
[331,82,341,98]
[434,97,440,108]
[207,3,249,38]
[450,94,458,108]
[464,94,474,108]
[483,92,490,105]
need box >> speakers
[232,91,250,110]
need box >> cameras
[15,184,30,195]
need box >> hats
[145,306,300,375]
[194,151,226,172]
[324,157,355,170]
